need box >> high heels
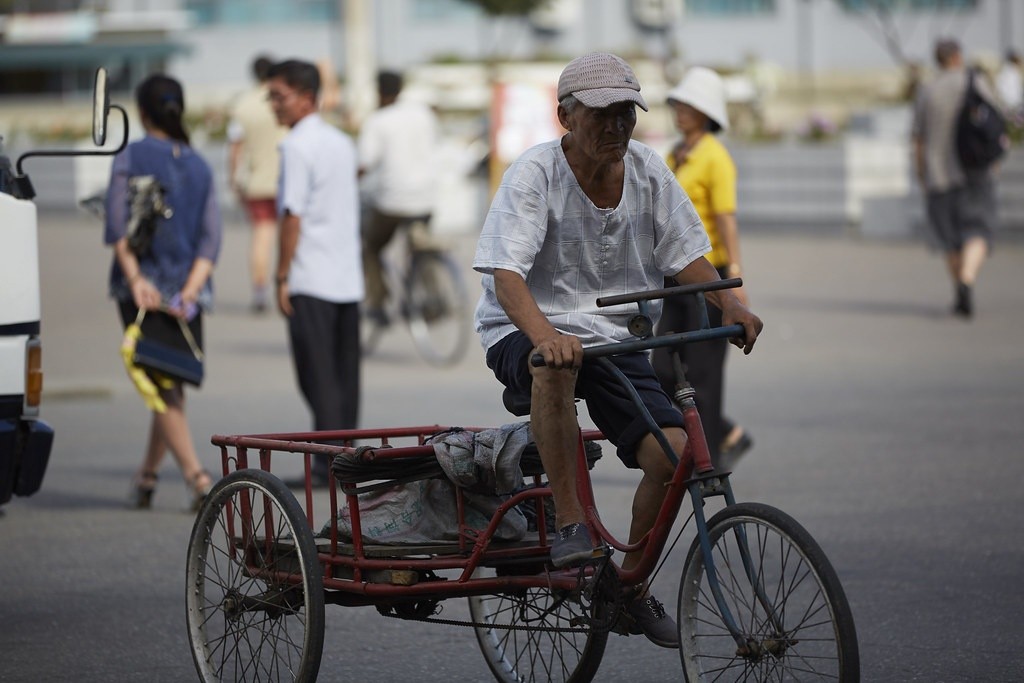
[130,471,159,509]
[186,469,214,515]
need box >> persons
[266,59,367,487]
[909,38,997,319]
[649,67,754,472]
[357,68,445,330]
[228,55,290,311]
[473,51,764,649]
[103,71,224,513]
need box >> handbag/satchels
[955,63,1010,173]
[120,171,164,262]
[132,303,204,386]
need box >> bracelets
[276,276,287,286]
[129,275,140,285]
[727,264,742,275]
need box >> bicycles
[364,216,473,366]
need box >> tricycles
[184,276,864,683]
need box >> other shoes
[955,278,974,319]
[622,594,680,648]
[286,470,330,490]
[721,421,752,475]
[549,523,594,567]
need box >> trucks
[0,66,132,516]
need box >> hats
[668,65,731,132]
[557,50,648,113]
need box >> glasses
[264,85,300,104]
